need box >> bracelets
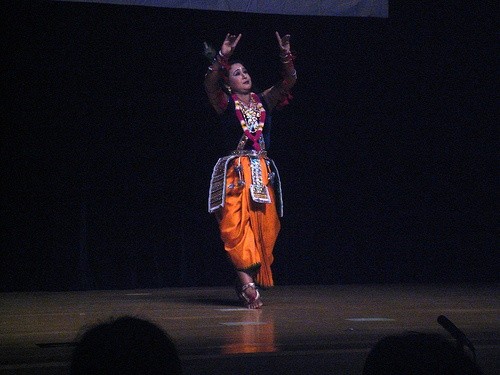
[281,52,295,64]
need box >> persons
[72,315,184,375]
[202,31,298,310]
[361,329,485,374]
